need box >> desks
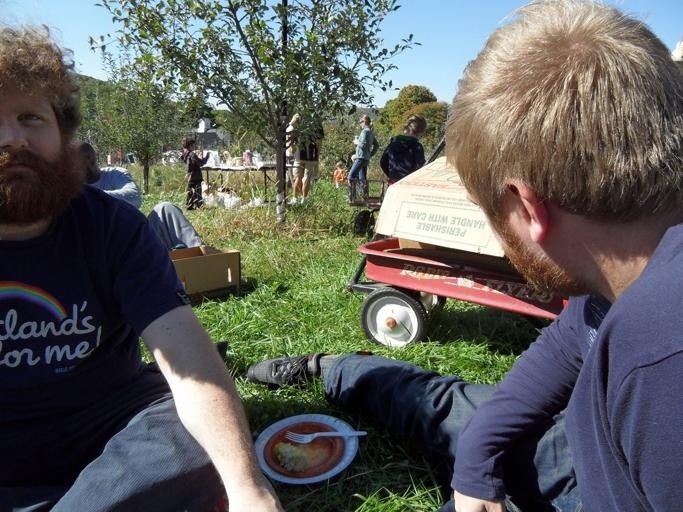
[201,165,298,202]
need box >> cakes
[272,441,306,471]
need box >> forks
[284,428,368,444]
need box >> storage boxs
[372,156,511,275]
[170,245,240,296]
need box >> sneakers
[247,352,322,386]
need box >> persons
[106,151,114,168]
[247,0,682,509]
[333,158,350,189]
[181,137,210,210]
[114,146,127,167]
[347,114,379,205]
[75,142,202,249]
[287,106,325,206]
[380,114,427,186]
[285,111,303,188]
[0,22,288,512]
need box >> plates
[256,413,360,484]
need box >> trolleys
[348,135,568,348]
